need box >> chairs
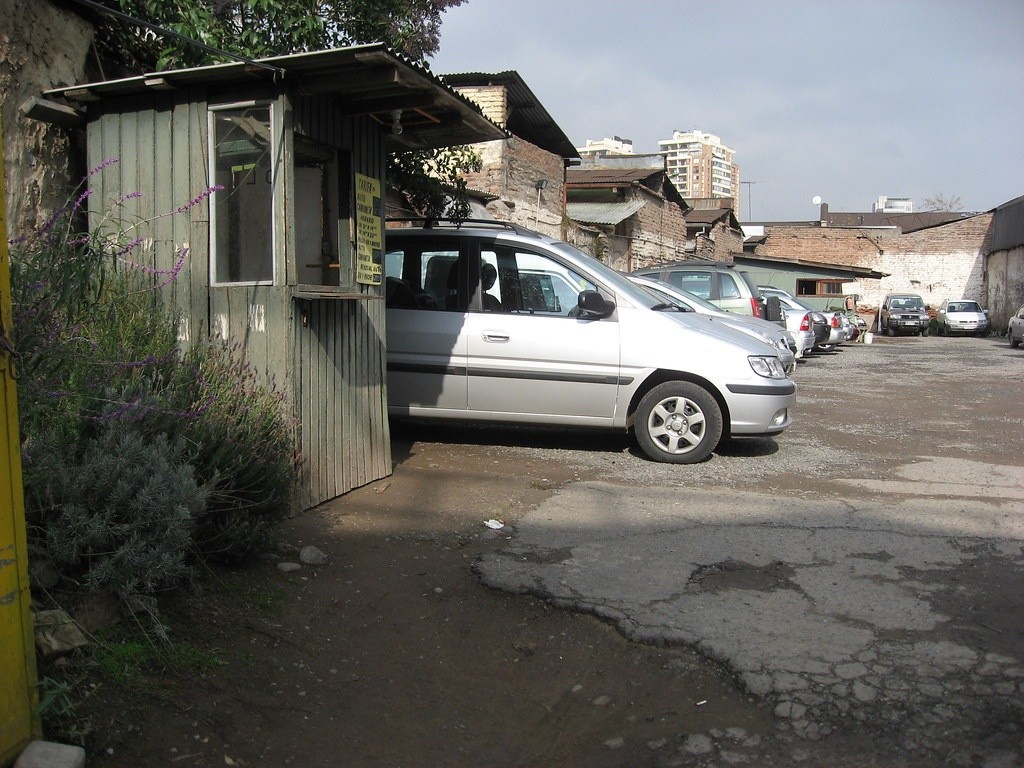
[481,263,501,311]
[445,261,457,310]
[892,300,899,304]
[949,306,955,310]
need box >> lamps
[534,180,548,190]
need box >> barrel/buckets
[864,332,873,344]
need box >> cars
[757,284,831,351]
[477,242,797,377]
[761,295,815,359]
[936,300,989,336]
[815,309,853,352]
[1008,303,1024,348]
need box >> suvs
[881,294,929,336]
[631,260,787,329]
[384,218,797,464]
